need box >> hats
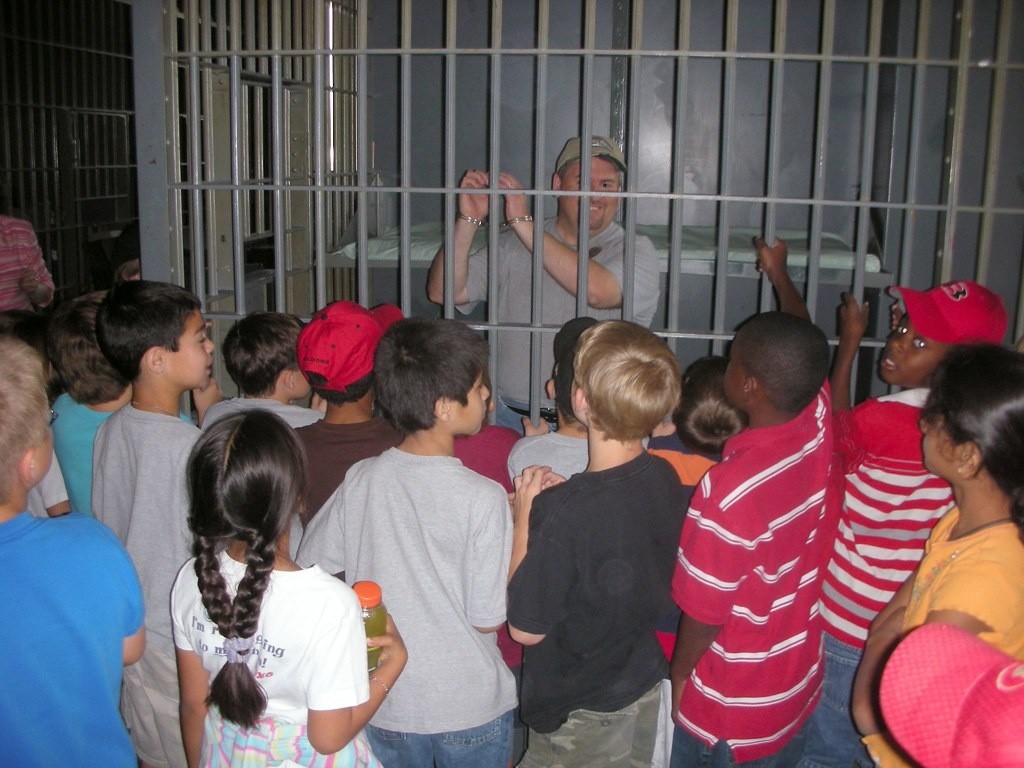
[297,300,406,395]
[554,317,599,415]
[556,136,627,174]
[878,623,1024,768]
[888,280,1008,347]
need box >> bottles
[351,581,388,670]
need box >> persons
[426,135,661,436]
[0,213,1024,768]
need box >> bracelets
[369,677,389,695]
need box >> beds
[316,219,894,296]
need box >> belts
[503,403,558,423]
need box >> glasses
[48,404,58,426]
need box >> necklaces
[130,400,179,417]
[951,517,1014,541]
